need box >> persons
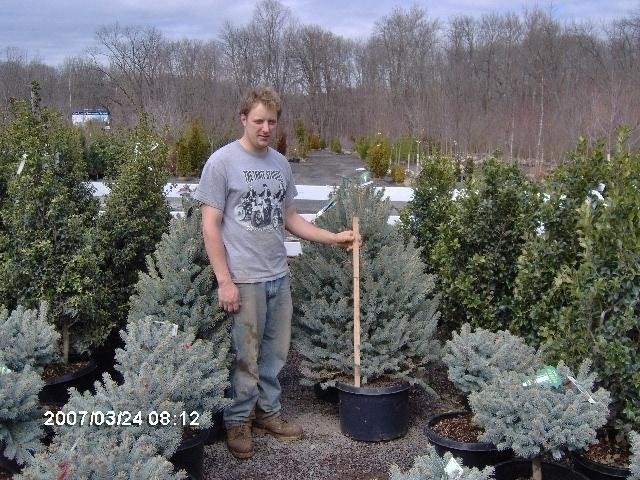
[193,86,363,459]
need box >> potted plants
[291,187,440,441]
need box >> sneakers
[253,419,304,441]
[227,422,254,458]
